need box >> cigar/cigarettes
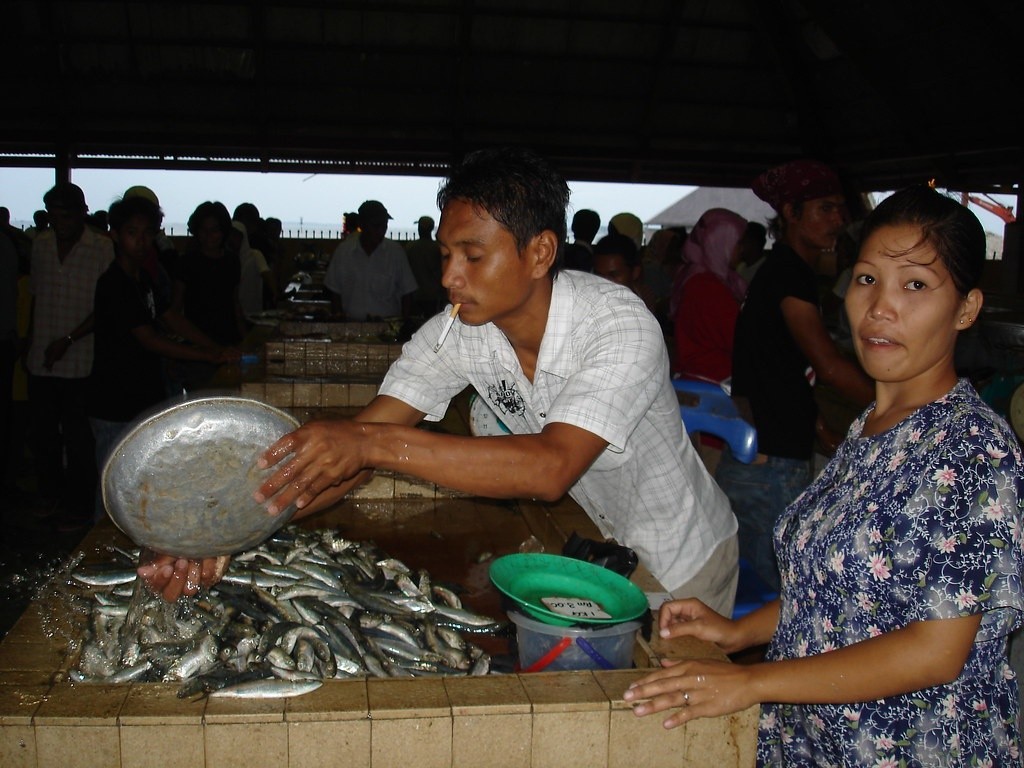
[434,304,461,353]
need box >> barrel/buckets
[507,609,645,673]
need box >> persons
[564,159,873,670]
[327,199,441,323]
[623,182,1024,768]
[136,148,740,655]
[0,182,321,534]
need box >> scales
[468,390,512,436]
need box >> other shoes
[58,517,92,533]
[34,502,57,518]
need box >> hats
[359,200,393,225]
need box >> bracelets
[65,335,74,343]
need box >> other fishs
[66,523,508,699]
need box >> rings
[679,689,690,706]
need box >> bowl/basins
[101,394,301,561]
[488,552,649,631]
